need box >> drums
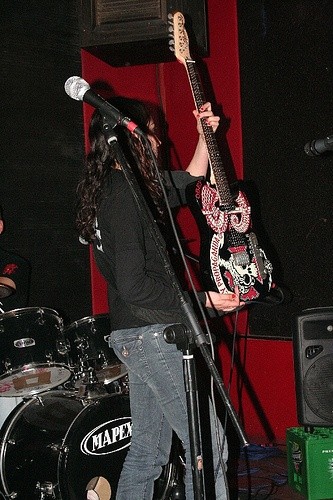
[65,315,127,389]
[0,387,179,500]
[0,308,75,398]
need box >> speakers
[291,308,332,434]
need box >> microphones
[304,133,333,157]
[64,74,145,141]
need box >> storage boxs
[286,425,333,500]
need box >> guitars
[168,11,273,306]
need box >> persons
[0,220,31,314]
[72,96,230,500]
